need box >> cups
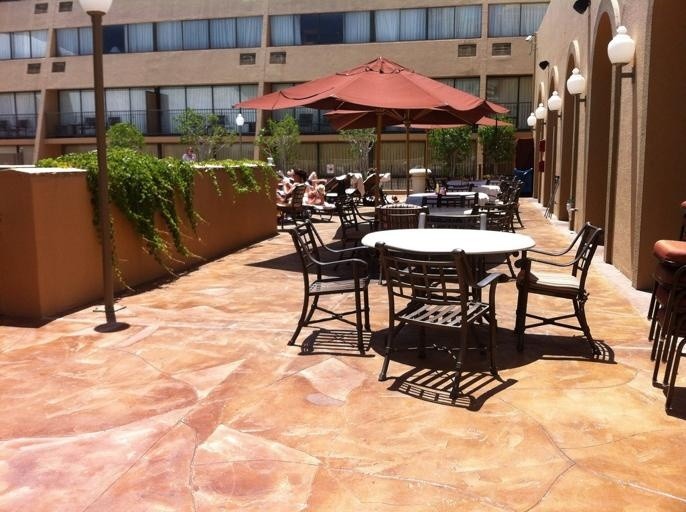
[266,157,274,165]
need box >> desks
[359,227,537,260]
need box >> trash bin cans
[409,168,432,193]
[514,168,533,197]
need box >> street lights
[234,111,246,161]
[77,0,130,333]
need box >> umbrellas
[393,116,514,177]
[232,55,511,230]
[323,109,473,197]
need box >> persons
[275,169,376,205]
[182,147,196,162]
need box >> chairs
[373,239,500,402]
[647,196,686,418]
[514,223,605,358]
[275,164,523,283]
[279,219,372,354]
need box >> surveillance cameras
[525,35,534,44]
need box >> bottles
[435,180,447,196]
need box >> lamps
[535,103,547,129]
[527,111,536,131]
[607,24,636,89]
[566,67,587,107]
[547,91,566,122]
